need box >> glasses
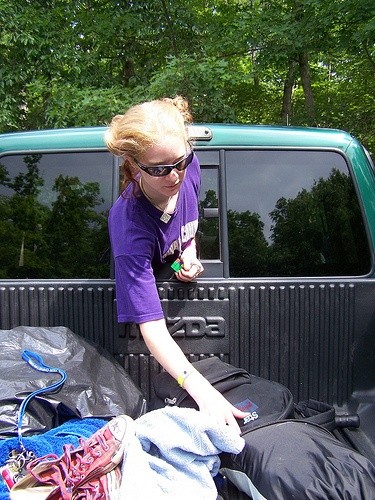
[131,141,194,177]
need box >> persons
[105,96,251,434]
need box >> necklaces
[140,175,175,224]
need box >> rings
[191,264,201,274]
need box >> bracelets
[176,366,194,389]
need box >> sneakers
[8,415,139,500]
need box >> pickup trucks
[0,123,375,465]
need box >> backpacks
[146,357,337,436]
[226,418,375,500]
